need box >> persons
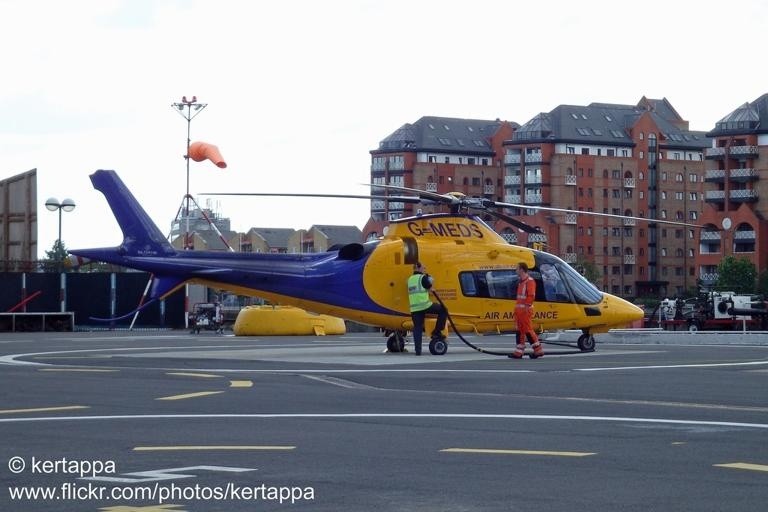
[506,261,543,360]
[406,259,447,358]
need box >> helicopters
[65,169,707,356]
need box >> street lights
[45,197,76,273]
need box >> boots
[507,344,526,359]
[528,342,544,359]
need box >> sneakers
[431,329,447,338]
[414,348,422,356]
